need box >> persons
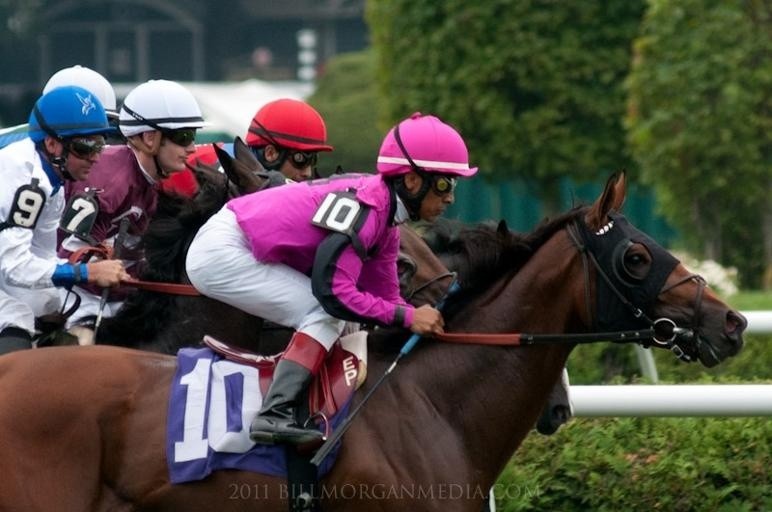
[184,110,480,446]
[1,64,335,356]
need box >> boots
[250,331,326,446]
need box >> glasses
[160,128,198,145]
[60,137,106,155]
[282,148,320,169]
[424,171,458,195]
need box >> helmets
[27,86,117,143]
[376,113,479,177]
[119,79,205,138]
[43,65,119,119]
[246,99,333,155]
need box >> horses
[0,136,749,511]
